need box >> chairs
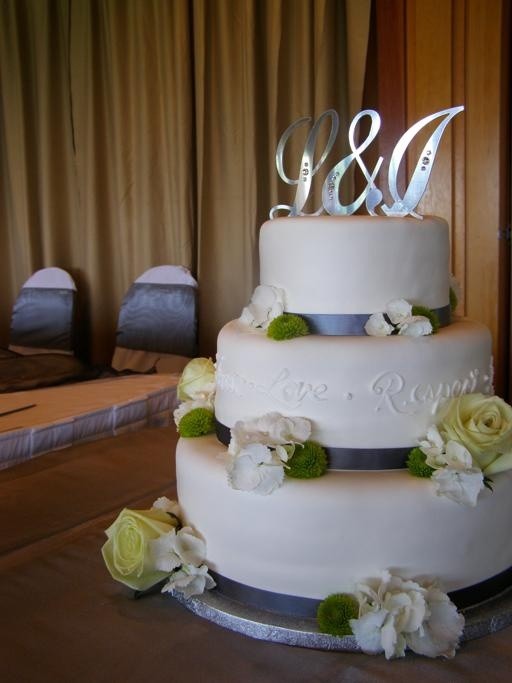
[111,263,201,391]
[0,266,77,400]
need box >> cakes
[174,104,512,622]
